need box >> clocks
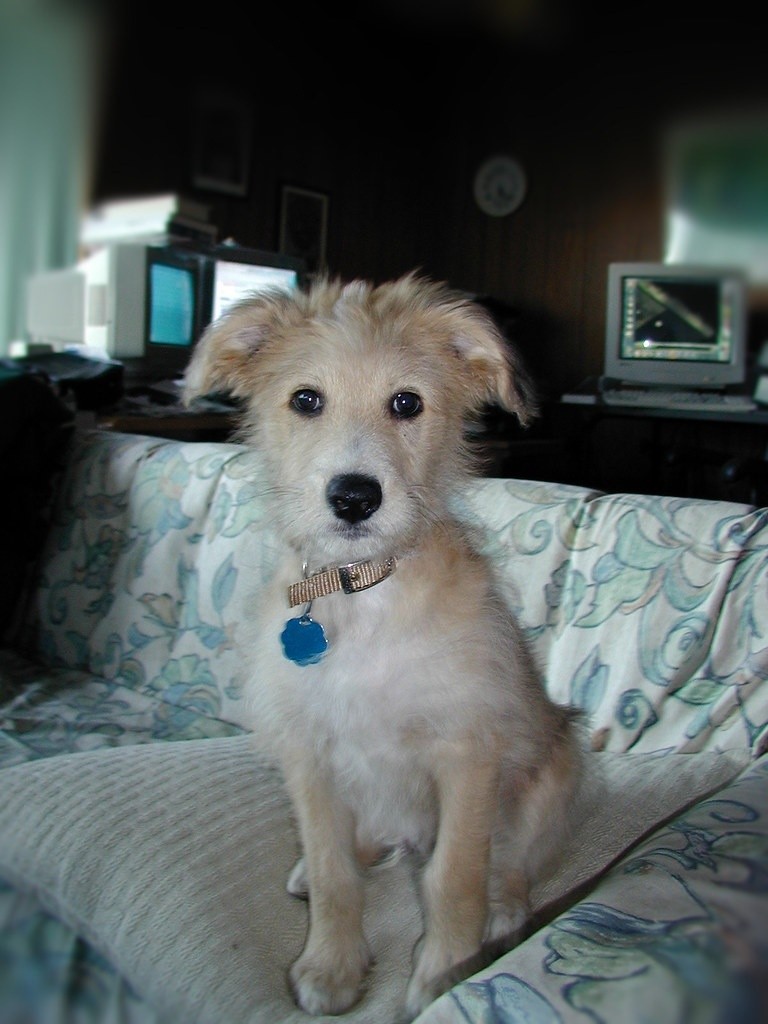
[474,157,527,217]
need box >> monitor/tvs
[605,263,744,389]
[28,232,308,392]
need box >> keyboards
[602,387,756,413]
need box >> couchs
[0,429,768,1024]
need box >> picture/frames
[273,180,332,273]
[184,83,254,204]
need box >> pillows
[0,732,753,1024]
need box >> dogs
[172,270,593,1017]
